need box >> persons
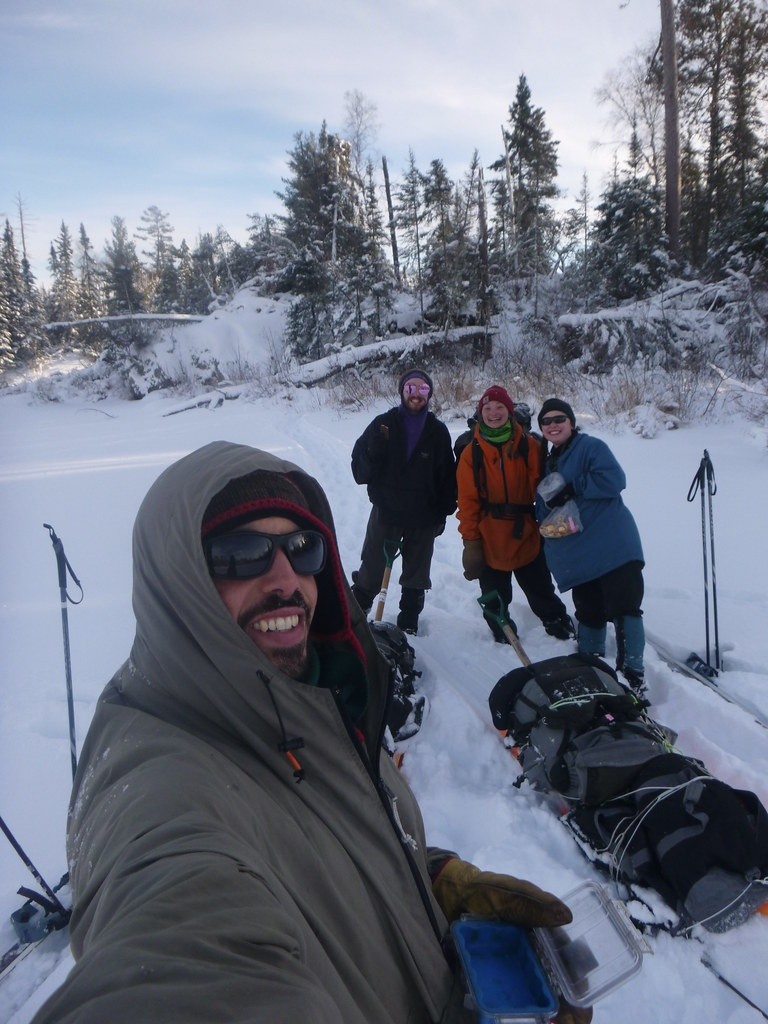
[350,369,457,635]
[455,385,577,644]
[28,440,594,1024]
[535,396,650,706]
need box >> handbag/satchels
[538,472,583,539]
[489,652,768,930]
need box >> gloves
[432,857,592,1024]
[462,539,485,581]
[547,487,575,509]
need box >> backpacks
[358,620,428,742]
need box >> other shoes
[555,621,576,641]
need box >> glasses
[200,529,328,580]
[403,382,431,395]
[540,415,569,425]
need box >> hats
[478,385,513,416]
[538,398,575,431]
[203,470,372,693]
[398,368,433,397]
